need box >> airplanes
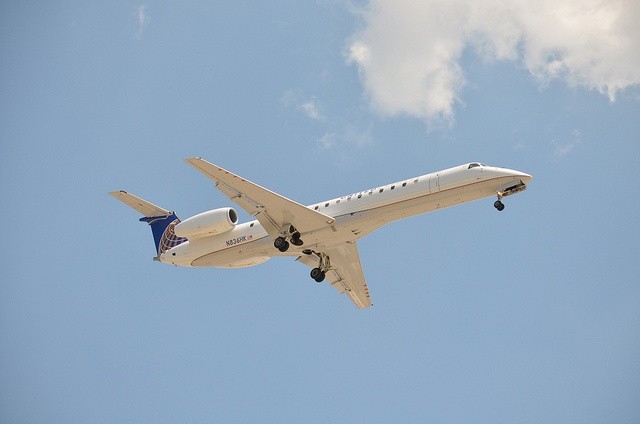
[108,156,532,310]
